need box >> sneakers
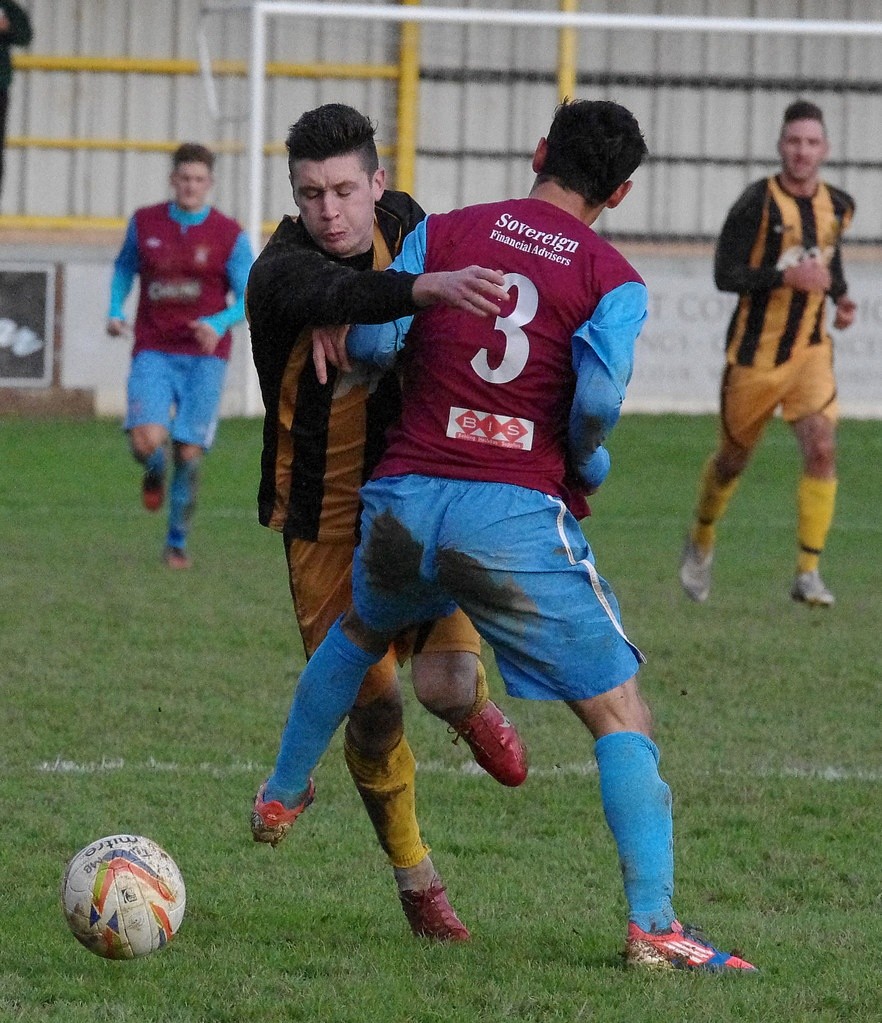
[398,875,469,941]
[250,777,315,847]
[791,569,834,606]
[679,536,714,603]
[447,698,527,787]
[624,919,760,975]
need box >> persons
[678,99,858,606]
[243,104,528,942]
[250,96,759,976]
[105,143,257,571]
[0,1,34,181]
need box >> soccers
[63,833,189,963]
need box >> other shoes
[165,549,191,571]
[140,472,165,508]
